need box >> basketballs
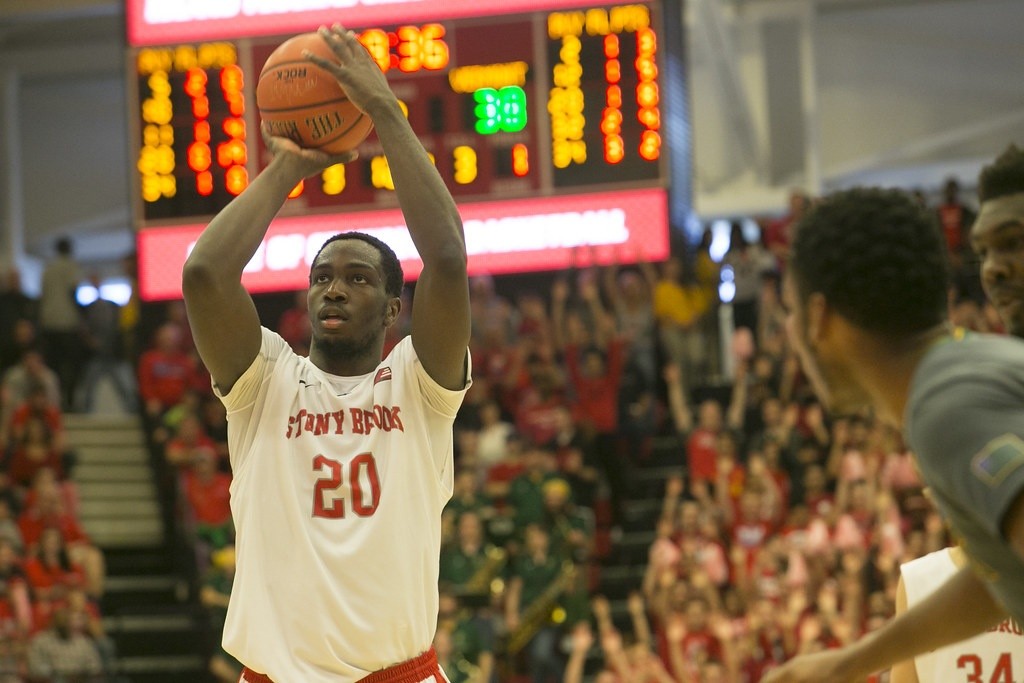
[256,30,382,155]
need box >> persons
[756,185,1024,683]
[147,176,1006,683]
[0,235,141,683]
[970,142,1024,342]
[180,24,469,683]
